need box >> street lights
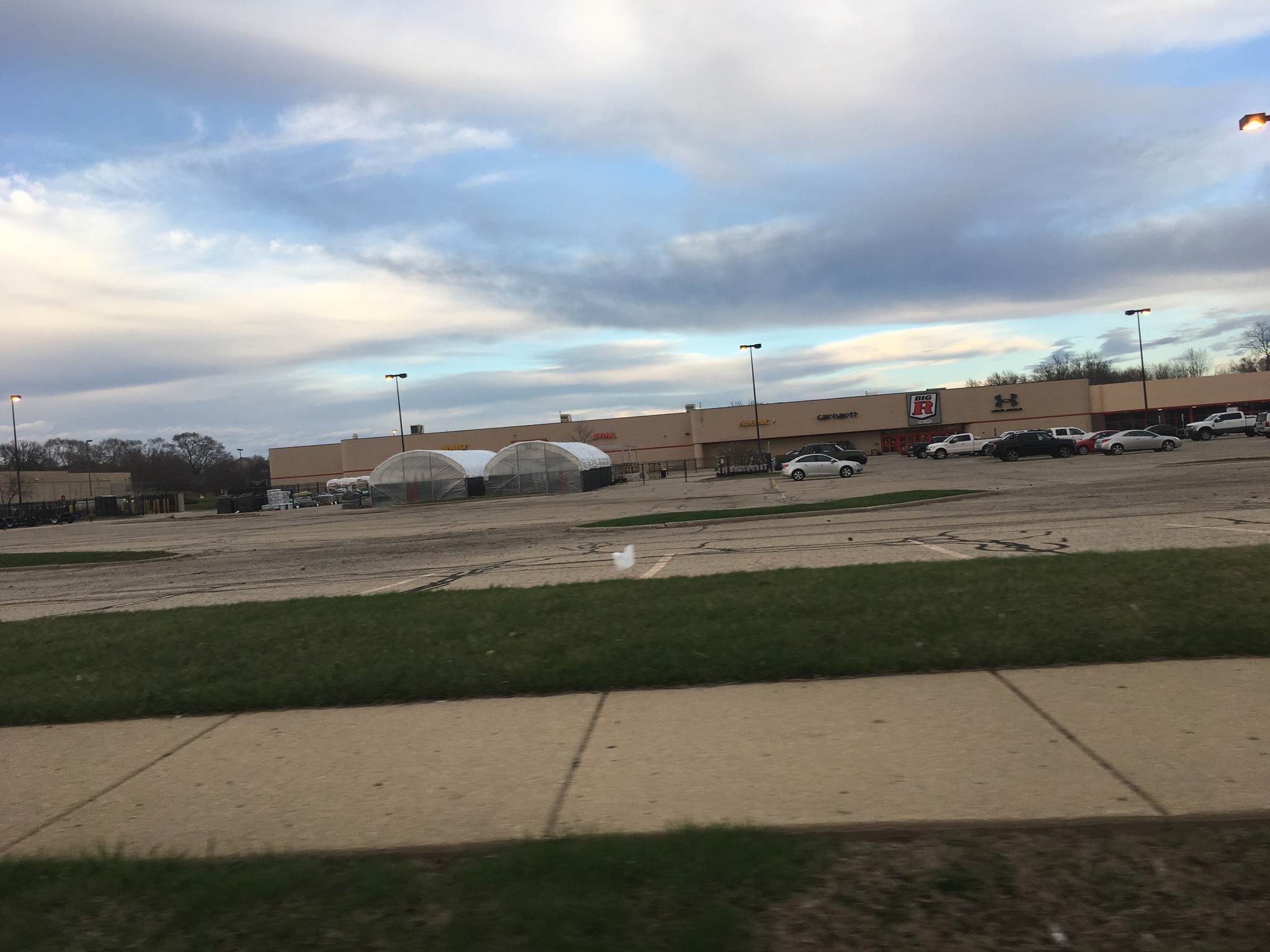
[237,448,243,469]
[1125,307,1152,429]
[385,373,408,452]
[739,343,766,465]
[86,440,93,497]
[10,394,23,510]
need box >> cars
[986,426,1088,462]
[780,453,863,481]
[771,442,868,471]
[1094,429,1182,456]
[1077,430,1122,455]
[1139,424,1187,440]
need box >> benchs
[660,464,674,470]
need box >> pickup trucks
[1185,411,1258,441]
[926,433,1002,460]
[906,435,948,459]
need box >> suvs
[1255,411,1270,439]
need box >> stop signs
[60,495,66,502]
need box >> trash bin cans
[661,470,666,478]
